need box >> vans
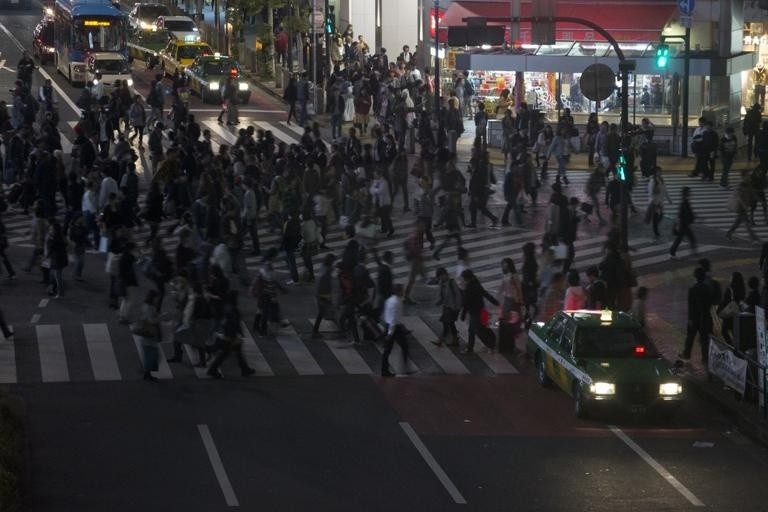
[87,52,133,98]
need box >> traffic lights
[656,44,670,70]
[326,13,336,35]
[617,146,634,185]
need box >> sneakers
[0,203,536,382]
[111,122,370,150]
[537,168,762,360]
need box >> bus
[55,1,133,86]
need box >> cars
[42,2,55,17]
[0,0,31,10]
[129,2,251,107]
[528,304,685,424]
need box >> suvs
[32,17,56,64]
